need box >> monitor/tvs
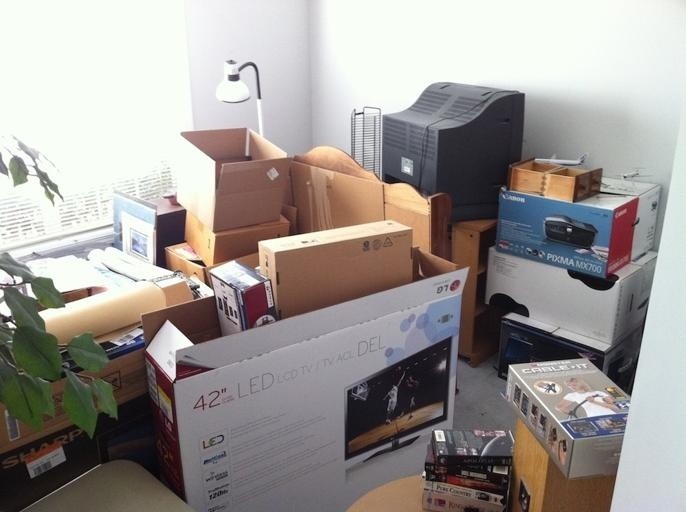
[382,81,526,224]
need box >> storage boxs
[173,128,291,233]
[166,241,230,289]
[211,259,278,336]
[495,172,660,279]
[483,243,659,347]
[141,245,470,511]
[111,190,187,271]
[257,220,413,318]
[497,313,644,397]
[183,206,292,269]
[504,356,632,479]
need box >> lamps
[215,57,264,138]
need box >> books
[422,430,515,512]
